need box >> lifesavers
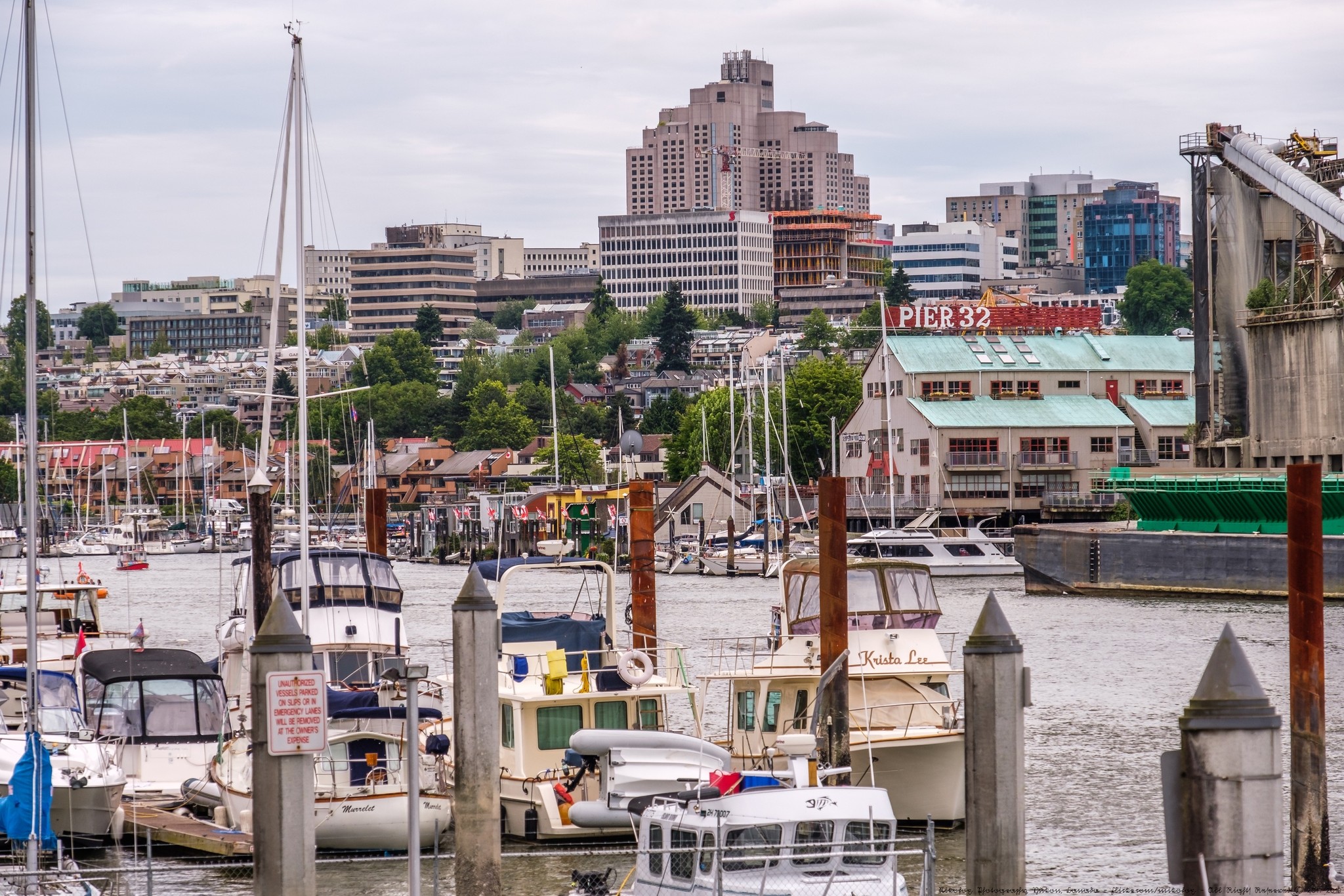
[77,575,88,584]
[617,649,654,685]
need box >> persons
[62,579,102,586]
[71,527,73,531]
[65,531,69,541]
[105,527,108,534]
[320,509,324,513]
[359,502,364,513]
[387,503,391,514]
[428,509,435,524]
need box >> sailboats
[0,1,262,867]
[0,290,1029,631]
[214,34,452,850]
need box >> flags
[131,622,143,646]
[479,461,483,472]
[510,503,590,522]
[607,504,617,524]
[73,631,86,657]
[79,562,82,573]
[398,519,411,531]
[35,569,41,584]
[452,505,497,522]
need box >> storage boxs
[740,774,780,792]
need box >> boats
[635,736,909,895]
[564,729,733,828]
[701,476,963,822]
[457,558,673,843]
[1010,515,1344,603]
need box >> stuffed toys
[351,405,358,422]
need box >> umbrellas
[430,457,434,467]
